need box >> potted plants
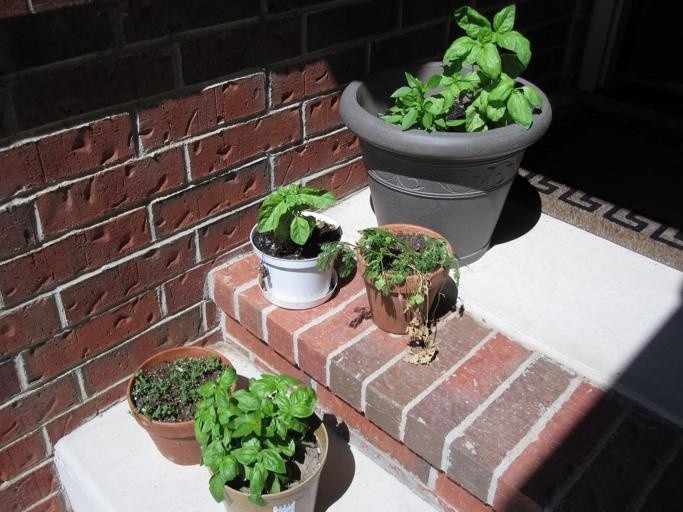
[127,345,331,511]
[250,183,452,335]
[340,5,554,266]
